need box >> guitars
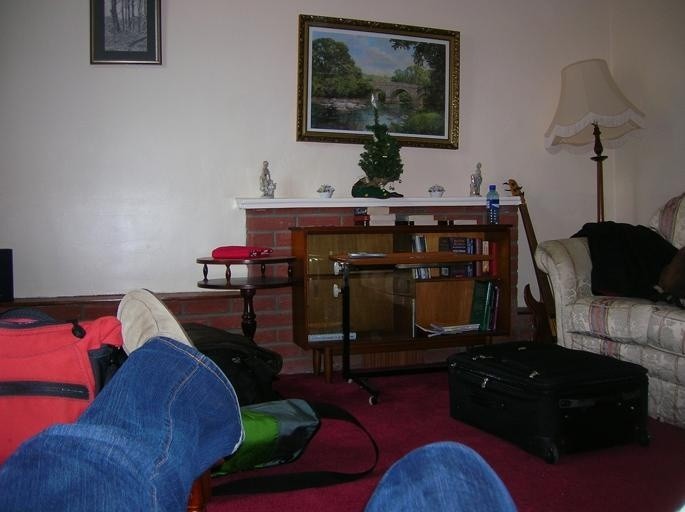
[502,179,557,343]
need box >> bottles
[486,185,499,224]
[471,163,482,196]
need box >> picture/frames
[89,0,162,66]
[295,13,464,150]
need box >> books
[413,233,502,335]
[354,204,479,227]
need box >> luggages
[447,339,651,462]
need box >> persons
[260,160,271,189]
[1,287,518,512]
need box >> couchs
[534,191,683,429]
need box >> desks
[197,250,296,343]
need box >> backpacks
[100,324,282,407]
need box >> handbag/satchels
[0,306,123,474]
[208,397,322,480]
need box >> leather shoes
[115,289,194,358]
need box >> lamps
[541,58,649,222]
